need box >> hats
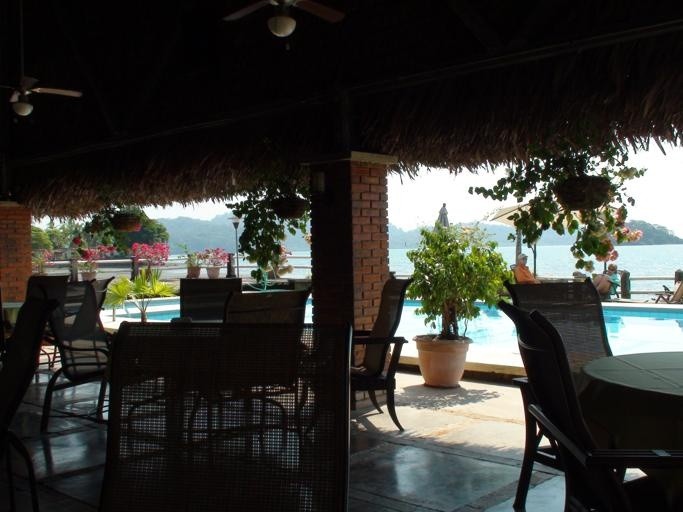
[518,252,528,260]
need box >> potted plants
[83,206,147,238]
[405,222,512,386]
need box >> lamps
[12,93,35,117]
[268,6,297,37]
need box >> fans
[1,1,84,124]
[225,0,345,38]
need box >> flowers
[475,159,645,273]
[129,242,170,269]
[71,236,117,271]
[177,242,229,265]
[271,199,307,217]
[226,191,310,273]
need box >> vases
[187,267,221,278]
[551,175,611,211]
[81,271,95,283]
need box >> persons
[591,263,620,301]
[657,269,682,303]
[513,253,540,284]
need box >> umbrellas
[489,195,618,228]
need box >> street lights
[226,215,244,279]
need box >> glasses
[608,267,616,272]
